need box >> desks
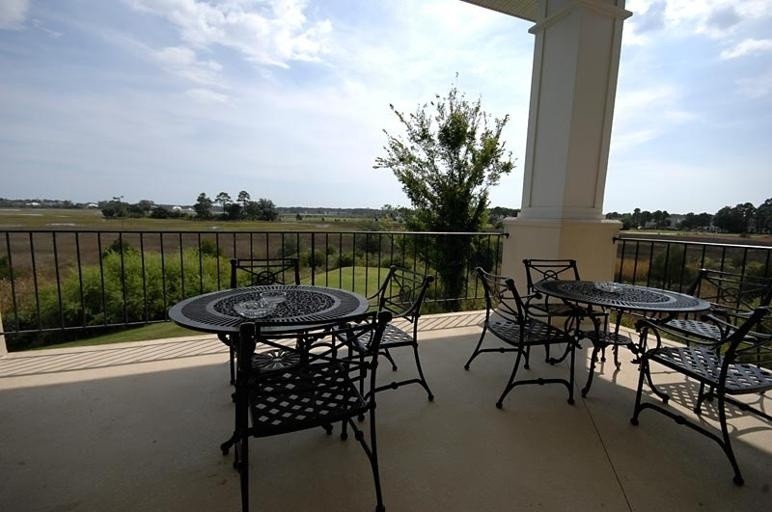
[168,284,369,456]
[530,275,712,409]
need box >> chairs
[632,265,769,414]
[628,302,770,486]
[520,259,608,373]
[229,259,303,384]
[468,266,580,413]
[331,264,436,422]
[234,313,392,511]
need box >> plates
[596,282,623,294]
[234,301,275,318]
